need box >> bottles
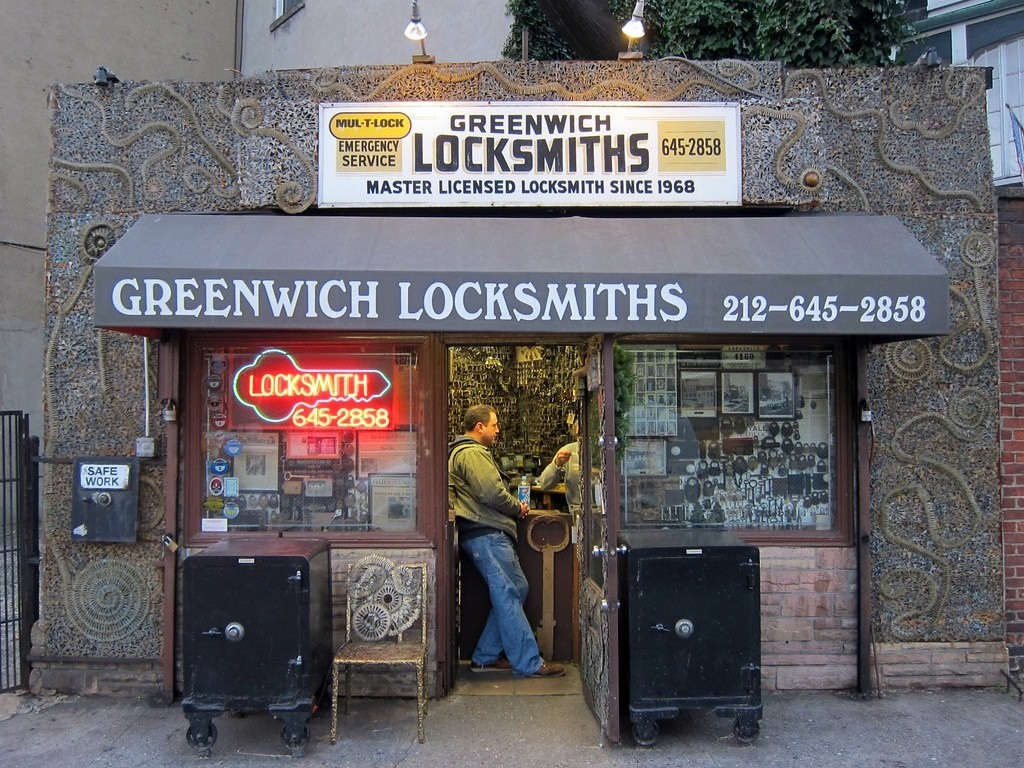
[518,476,530,510]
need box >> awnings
[94,213,951,338]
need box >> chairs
[329,553,429,745]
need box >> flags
[1010,111,1024,186]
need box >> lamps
[404,0,435,63]
[619,0,646,59]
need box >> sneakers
[528,661,565,678]
[470,656,512,672]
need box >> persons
[449,405,566,678]
[540,442,582,519]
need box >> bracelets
[552,459,561,469]
